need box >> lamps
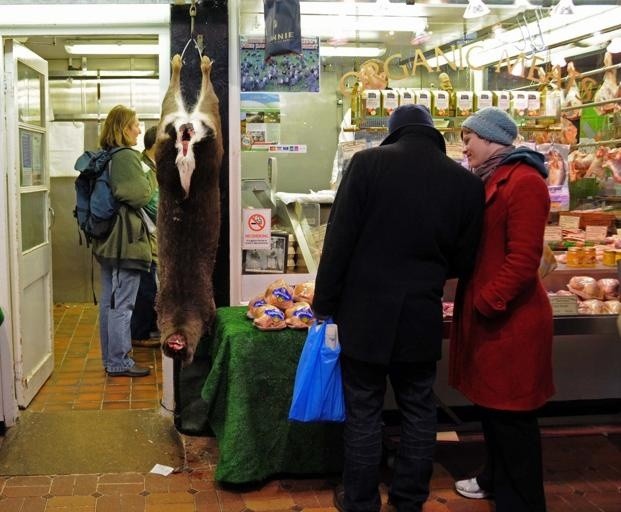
[63,36,158,59]
[463,0,489,19]
[320,42,389,59]
[549,0,576,16]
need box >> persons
[93,103,153,378]
[309,102,488,512]
[445,107,555,512]
[128,124,162,348]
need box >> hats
[387,102,436,133]
[460,106,519,146]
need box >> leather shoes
[107,365,152,377]
[331,485,346,512]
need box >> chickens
[557,276,621,316]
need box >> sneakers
[130,337,161,348]
[454,476,486,498]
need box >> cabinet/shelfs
[241,179,316,303]
[293,201,621,411]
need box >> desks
[216,306,346,485]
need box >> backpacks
[71,145,137,249]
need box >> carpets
[1,408,186,476]
